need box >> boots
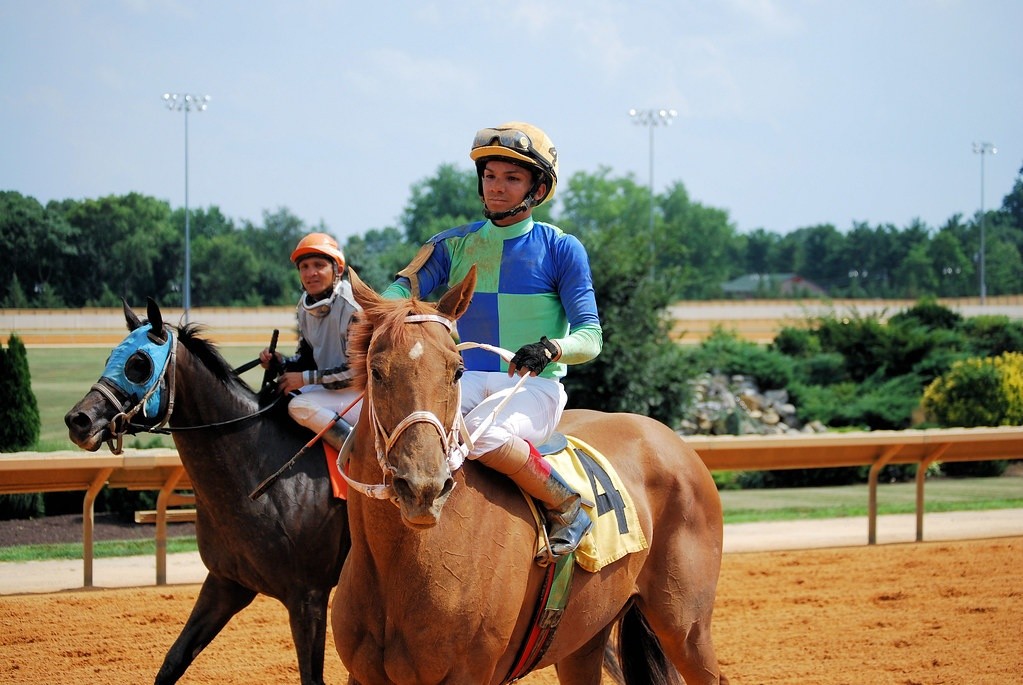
[322,412,354,452]
[507,439,594,555]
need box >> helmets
[469,121,559,220]
[290,232,345,289]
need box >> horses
[331,263,728,685]
[64,295,352,685]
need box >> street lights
[163,92,215,325]
[971,142,999,303]
[630,108,678,278]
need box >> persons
[380,122,602,560]
[259,233,367,458]
